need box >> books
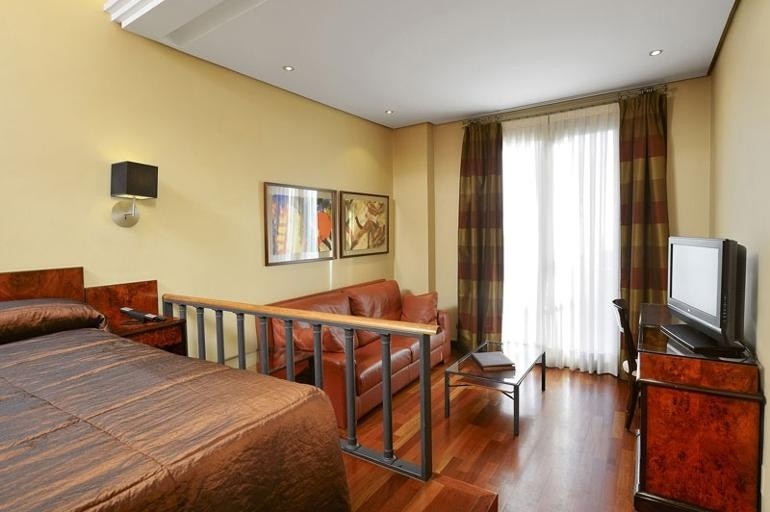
[470,350,516,372]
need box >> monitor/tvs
[659,235,747,354]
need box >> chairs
[612,299,641,431]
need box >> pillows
[293,326,345,352]
[0,298,106,345]
[400,291,438,325]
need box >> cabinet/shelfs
[215,343,315,388]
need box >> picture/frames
[264,182,337,266]
[339,190,389,259]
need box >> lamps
[111,161,158,228]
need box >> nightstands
[84,280,187,355]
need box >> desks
[634,304,766,512]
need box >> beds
[0,267,349,512]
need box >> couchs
[256,279,451,430]
